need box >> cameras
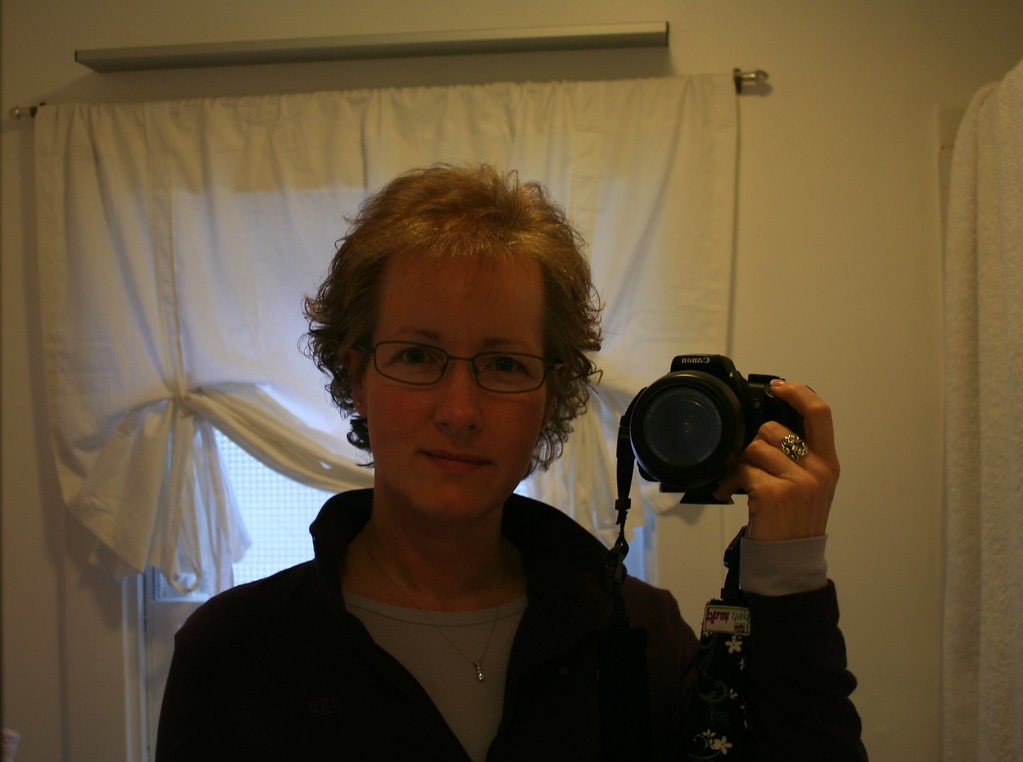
[631,353,805,505]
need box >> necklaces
[360,534,506,681]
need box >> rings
[781,435,809,463]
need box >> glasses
[367,340,551,394]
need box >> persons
[155,164,868,762]
